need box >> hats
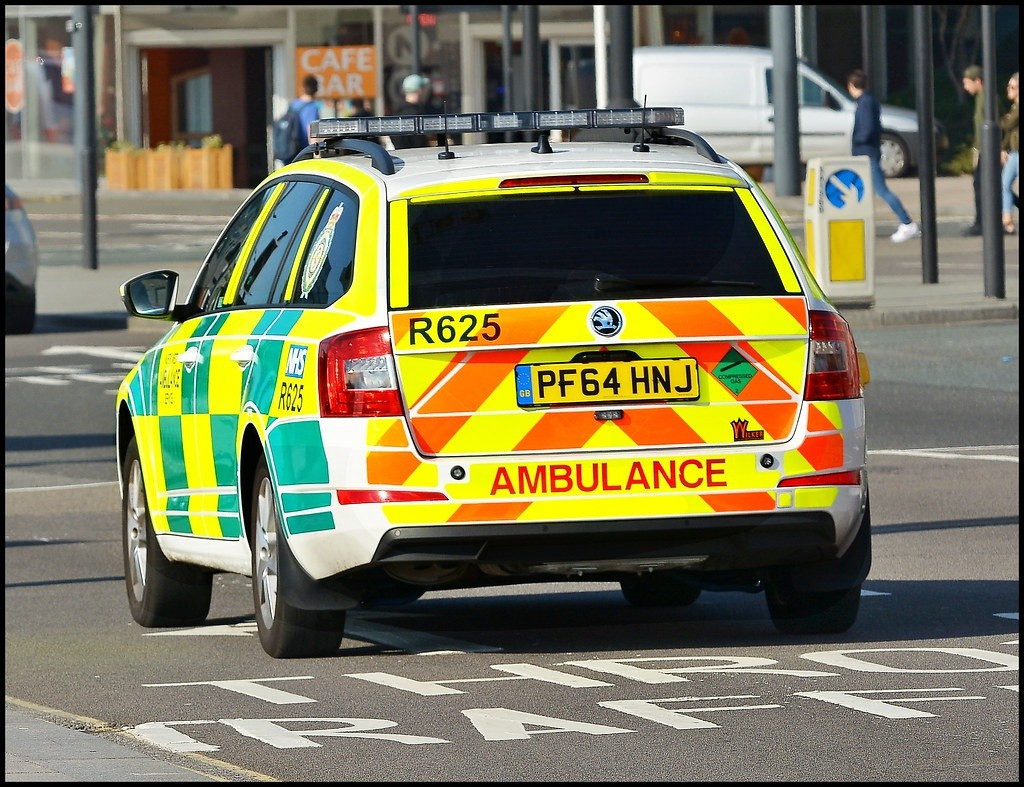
[402,74,430,92]
[962,66,983,78]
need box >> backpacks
[272,101,313,160]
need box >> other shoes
[963,222,983,236]
[1002,221,1017,233]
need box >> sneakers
[890,222,922,243]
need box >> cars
[6,186,37,334]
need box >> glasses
[1007,83,1019,91]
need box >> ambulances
[114,107,872,659]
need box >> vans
[632,45,949,177]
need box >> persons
[349,98,372,117]
[999,71,1018,233]
[283,75,320,166]
[963,64,986,235]
[383,74,431,149]
[848,70,919,241]
